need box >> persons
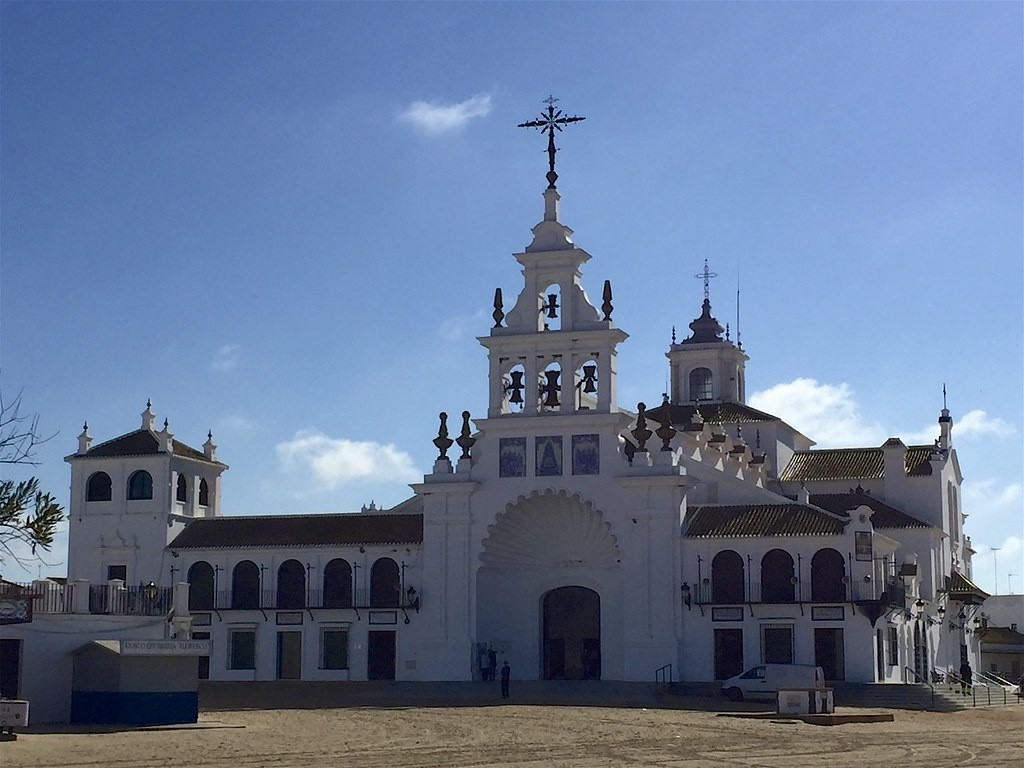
[960,660,972,697]
[480,649,497,681]
[501,661,510,699]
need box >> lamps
[912,597,927,620]
[680,581,692,611]
[407,585,420,614]
[970,617,980,635]
[928,605,946,627]
[948,611,968,632]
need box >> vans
[721,663,826,703]
